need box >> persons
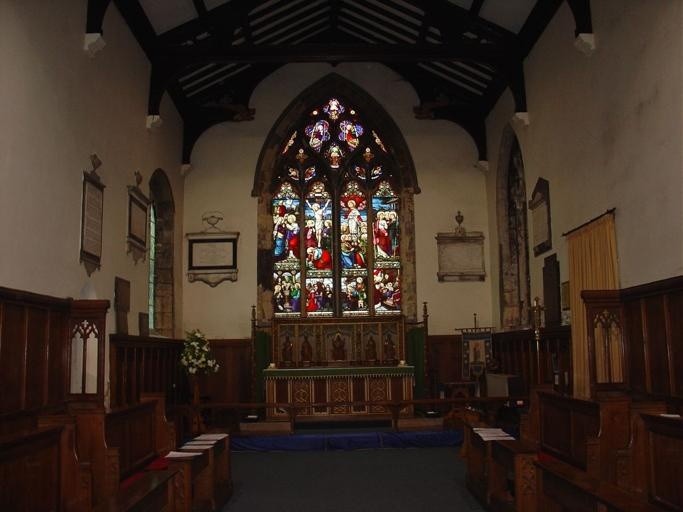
[281,334,292,361]
[300,334,312,361]
[365,335,376,361]
[271,200,401,311]
[330,334,346,361]
[383,334,395,361]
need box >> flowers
[178,328,220,380]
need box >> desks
[89,433,233,512]
[461,419,664,512]
